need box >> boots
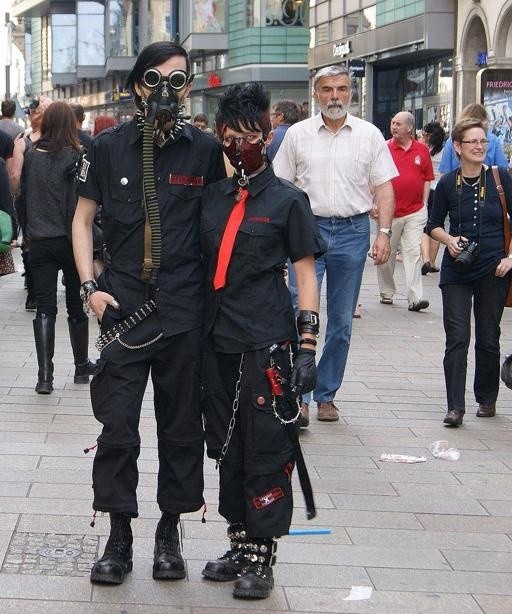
[202,525,248,581]
[154,516,186,580]
[90,515,133,583]
[67,318,97,384]
[234,537,277,598]
[32,316,54,394]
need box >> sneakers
[317,402,339,421]
[300,400,310,427]
[25,295,37,313]
[408,298,429,311]
[380,297,393,304]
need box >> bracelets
[300,339,317,346]
[79,279,97,301]
[296,310,319,334]
[506,255,511,258]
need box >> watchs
[379,228,392,237]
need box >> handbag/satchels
[0,211,12,253]
[504,269,512,307]
[68,145,103,253]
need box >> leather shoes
[422,262,431,276]
[477,403,496,417]
[431,267,439,272]
[444,409,463,426]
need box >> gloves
[101,245,113,271]
[289,348,317,394]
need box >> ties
[214,191,248,291]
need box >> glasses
[460,140,490,147]
[142,70,188,88]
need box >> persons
[418,122,449,274]
[91,116,117,137]
[422,119,511,424]
[0,100,47,308]
[437,103,508,175]
[70,103,95,153]
[369,112,430,311]
[262,99,300,167]
[194,80,331,601]
[15,101,98,395]
[269,66,400,424]
[69,40,230,585]
[193,113,208,132]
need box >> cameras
[454,240,479,275]
